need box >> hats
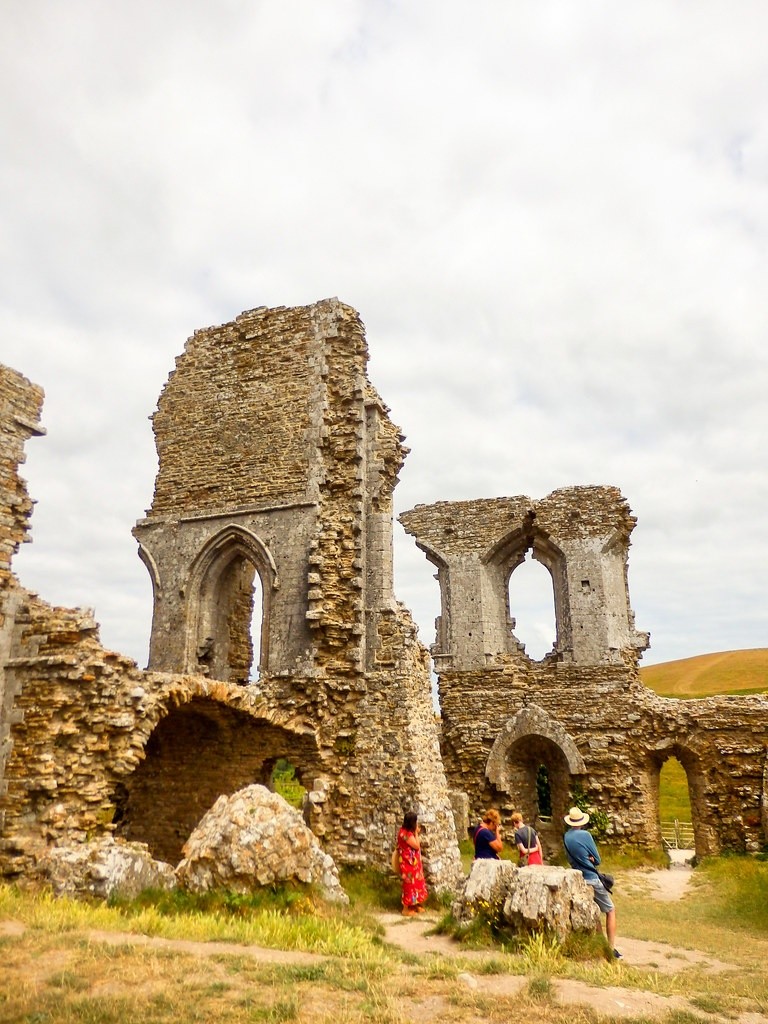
[564,807,590,826]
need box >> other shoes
[610,949,624,958]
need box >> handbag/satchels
[517,854,529,866]
[600,873,614,889]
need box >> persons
[562,806,625,959]
[467,810,503,861]
[396,812,429,916]
[511,813,544,865]
